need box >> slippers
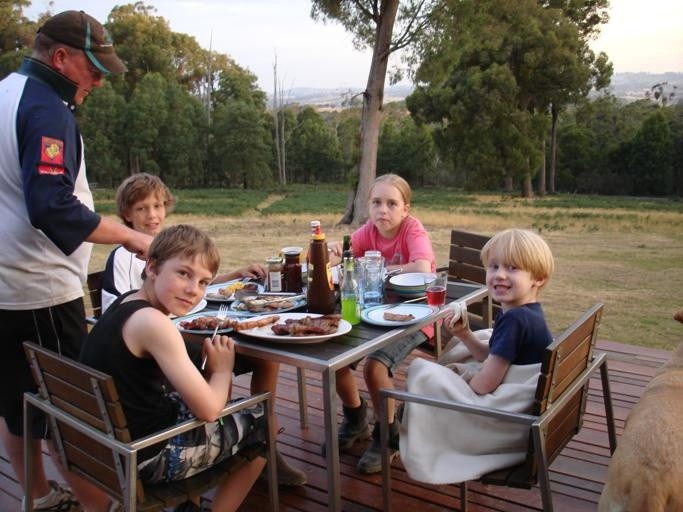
[390,448,408,473]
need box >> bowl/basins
[390,272,437,299]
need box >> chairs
[23,341,278,511]
[86,271,113,326]
[379,302,618,512]
[415,227,502,361]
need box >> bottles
[281,246,303,293]
[266,256,286,293]
[307,225,336,314]
[341,250,361,326]
[306,220,321,275]
[341,235,353,271]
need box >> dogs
[598,308,683,512]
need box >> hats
[39,10,131,77]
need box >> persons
[402,228,556,485]
[83,222,280,510]
[0,10,156,512]
[326,172,437,475]
[97,171,271,316]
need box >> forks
[200,304,229,371]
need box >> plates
[160,299,207,319]
[227,295,307,315]
[360,304,440,327]
[175,312,242,334]
[204,282,266,303]
[236,313,353,344]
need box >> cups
[425,272,448,307]
[358,250,385,306]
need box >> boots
[321,401,372,456]
[357,420,401,473]
[251,443,309,489]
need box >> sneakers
[22,480,79,512]
[174,499,211,512]
